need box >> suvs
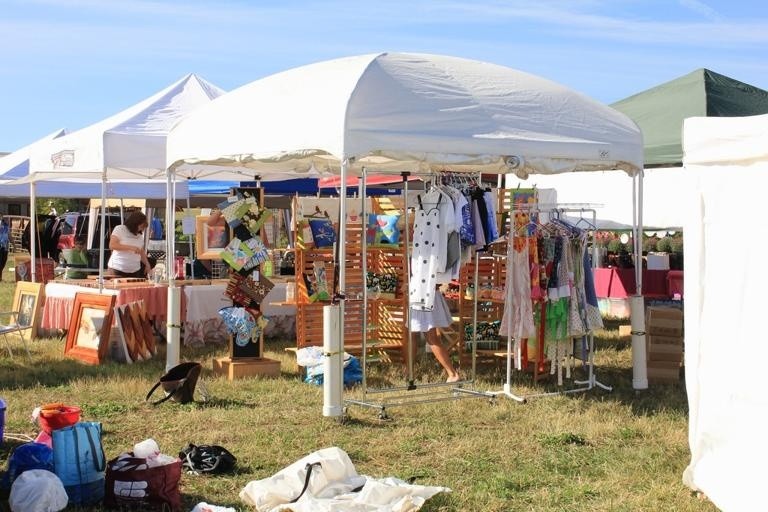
[46,209,124,266]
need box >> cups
[147,269,165,283]
[111,279,120,290]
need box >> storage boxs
[644,303,685,339]
[644,335,686,364]
[646,359,683,386]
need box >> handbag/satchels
[463,318,502,352]
[304,357,363,387]
[51,422,106,505]
[179,444,236,474]
[105,453,181,512]
[146,362,201,406]
[608,251,683,271]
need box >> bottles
[59,258,64,268]
[280,257,294,274]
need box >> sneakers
[446,373,461,383]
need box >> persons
[207,212,270,268]
[397,214,462,382]
[0,212,16,281]
[64,236,88,279]
[107,211,151,280]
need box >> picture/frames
[8,279,45,341]
[194,216,231,261]
[63,291,118,369]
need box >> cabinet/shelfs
[268,187,532,374]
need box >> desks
[42,274,166,355]
[183,272,295,351]
[582,265,667,323]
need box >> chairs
[0,306,32,361]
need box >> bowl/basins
[38,407,81,436]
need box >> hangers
[503,203,599,242]
[410,171,480,210]
